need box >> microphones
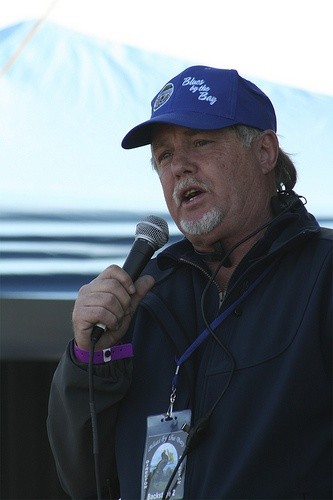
[90,215,170,343]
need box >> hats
[121,66,277,150]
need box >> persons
[47,65,333,500]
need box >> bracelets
[73,340,134,365]
